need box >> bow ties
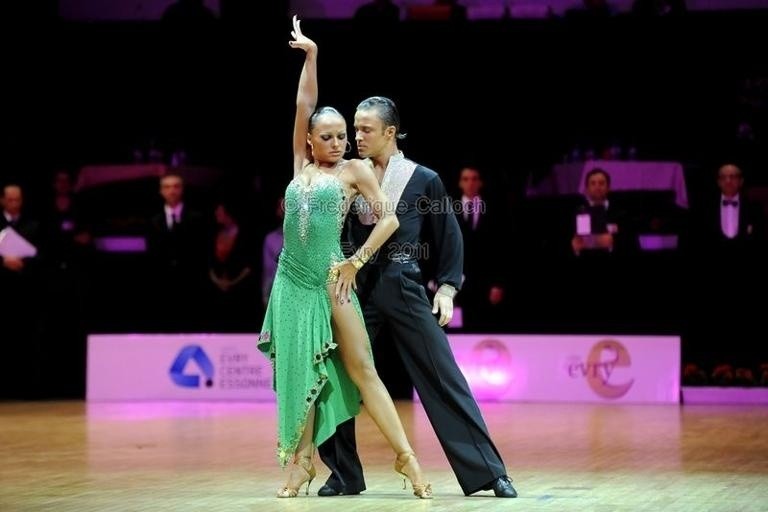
[723,199,738,207]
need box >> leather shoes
[318,484,340,495]
[491,475,517,498]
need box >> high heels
[277,456,316,498]
[395,451,434,499]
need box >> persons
[30,167,100,368]
[136,171,213,333]
[0,184,57,401]
[676,159,767,388]
[255,14,434,498]
[439,162,519,332]
[200,196,255,330]
[256,196,291,308]
[318,95,520,498]
[553,164,649,335]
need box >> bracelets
[348,249,371,269]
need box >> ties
[172,213,178,227]
[466,202,474,229]
